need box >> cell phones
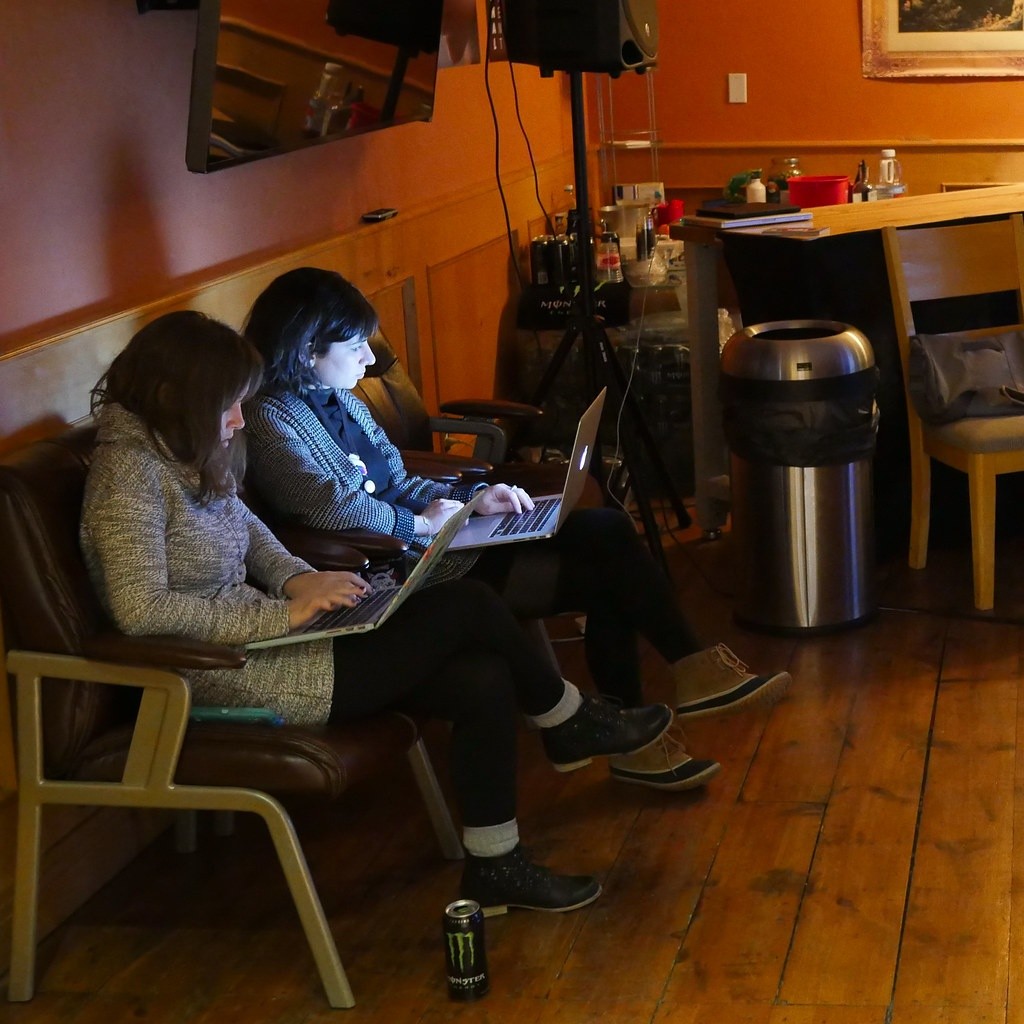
[361,209,399,223]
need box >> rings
[511,485,517,491]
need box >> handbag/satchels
[908,331,1024,424]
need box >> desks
[512,278,688,354]
[668,182,1024,542]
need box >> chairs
[880,213,1024,609]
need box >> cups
[651,200,685,226]
[594,242,624,283]
[635,216,655,260]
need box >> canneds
[530,234,571,284]
[601,231,620,259]
[443,899,490,1001]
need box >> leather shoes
[460,843,602,918]
[542,691,673,772]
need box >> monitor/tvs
[186,0,444,175]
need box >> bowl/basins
[626,260,666,286]
[619,241,684,262]
[787,175,848,209]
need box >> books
[682,199,809,229]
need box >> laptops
[243,490,488,649]
[444,387,608,552]
[682,213,812,228]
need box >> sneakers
[607,732,720,792]
[666,642,792,719]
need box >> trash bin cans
[721,321,876,633]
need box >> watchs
[419,516,431,534]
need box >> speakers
[499,0,659,79]
[324,0,443,58]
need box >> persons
[243,266,793,791]
[78,309,676,912]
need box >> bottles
[746,157,803,207]
[345,85,363,130]
[851,149,906,203]
[305,62,346,132]
[555,185,576,235]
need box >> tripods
[504,69,696,589]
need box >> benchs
[0,324,604,1010]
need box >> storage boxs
[786,174,850,208]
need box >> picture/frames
[861,0,1024,79]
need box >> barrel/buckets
[598,203,649,237]
